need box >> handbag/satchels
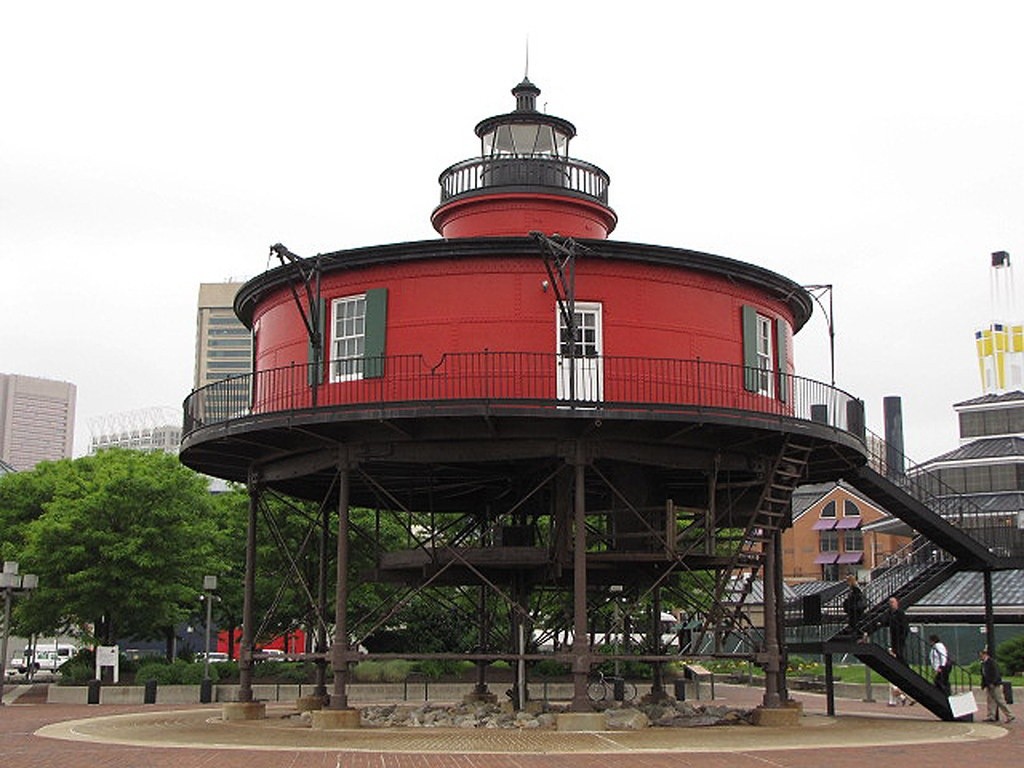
[941,661,952,674]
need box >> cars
[254,649,287,664]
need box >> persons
[844,575,868,645]
[888,648,906,707]
[978,650,1016,724]
[928,635,952,698]
[889,597,910,665]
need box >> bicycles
[587,670,637,703]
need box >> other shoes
[902,699,906,705]
[983,717,995,722]
[1005,717,1015,724]
[909,701,915,706]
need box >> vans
[195,652,229,663]
[11,644,81,676]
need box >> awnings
[813,552,839,566]
[835,518,862,529]
[812,519,838,530]
[836,552,864,565]
